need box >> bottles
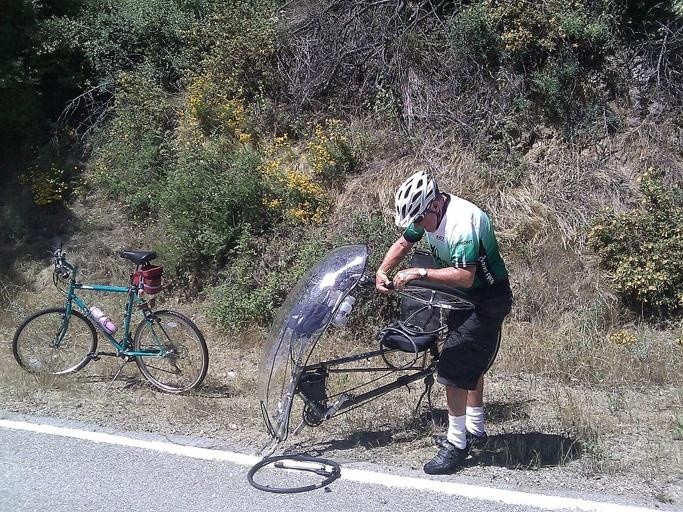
[89,305,117,335]
[335,297,354,327]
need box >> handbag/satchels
[477,288,513,315]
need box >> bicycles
[14,241,209,394]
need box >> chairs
[381,250,441,353]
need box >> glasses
[414,198,436,223]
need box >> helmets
[396,171,440,227]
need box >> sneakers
[437,427,488,447]
[424,439,468,474]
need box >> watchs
[418,268,427,280]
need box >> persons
[374,170,514,475]
